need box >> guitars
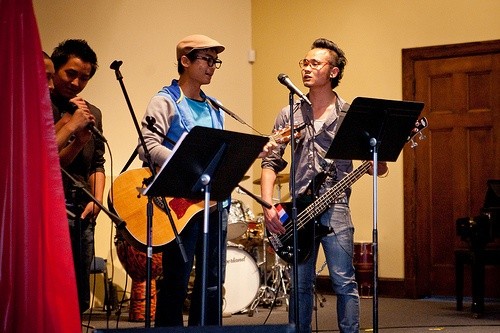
[266,117,428,265]
[107,123,302,254]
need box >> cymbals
[253,174,290,184]
[240,176,250,181]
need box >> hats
[176,34,225,61]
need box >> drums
[227,199,248,241]
[240,219,262,239]
[115,240,162,322]
[185,245,262,317]
[353,243,373,299]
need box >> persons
[259,36,390,332]
[42,51,55,92]
[137,33,233,332]
[50,36,108,315]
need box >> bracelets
[377,167,389,178]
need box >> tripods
[249,217,327,317]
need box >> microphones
[67,103,107,143]
[278,74,312,106]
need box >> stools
[456,248,500,317]
[91,257,112,315]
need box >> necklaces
[312,101,333,109]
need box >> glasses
[298,59,335,70]
[190,56,222,69]
